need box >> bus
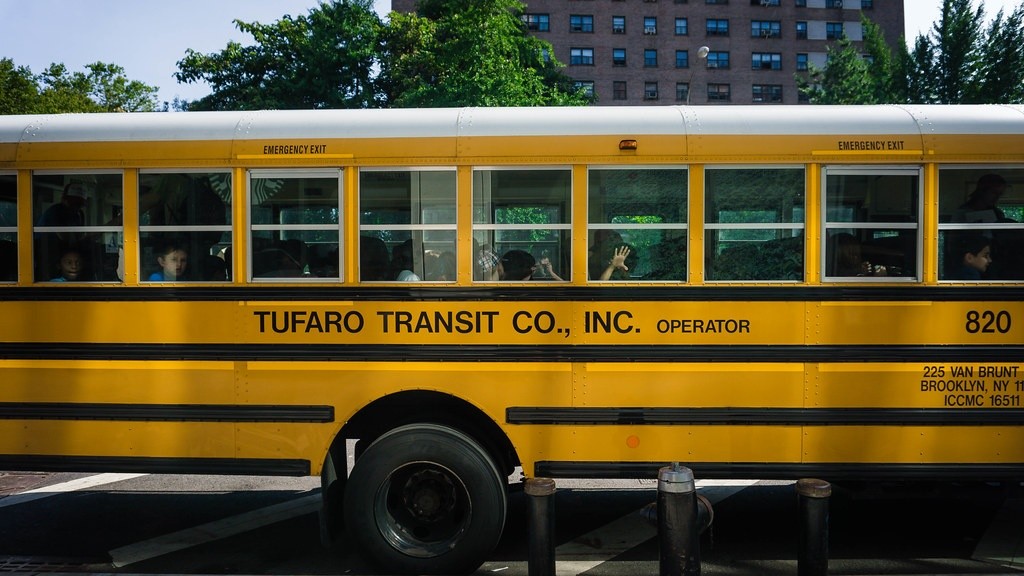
[1,103,1024,576]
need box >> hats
[588,229,623,251]
[63,183,83,200]
[965,173,1006,209]
[273,239,305,270]
[475,244,500,276]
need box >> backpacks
[163,174,226,246]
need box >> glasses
[398,255,413,265]
[313,266,338,277]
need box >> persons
[48,247,87,282]
[35,173,1024,282]
[147,245,188,283]
